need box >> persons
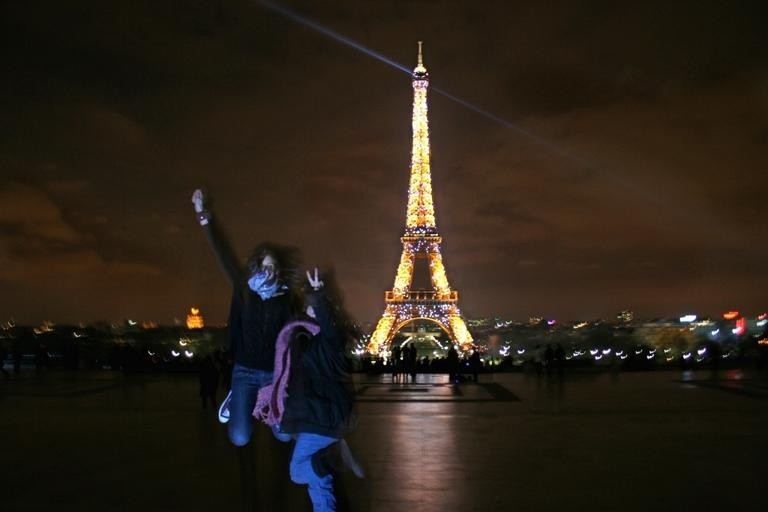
[359,342,565,383]
[191,190,302,448]
[0,335,234,408]
[251,264,367,512]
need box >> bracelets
[193,211,211,222]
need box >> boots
[310,439,364,482]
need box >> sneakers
[218,390,233,423]
